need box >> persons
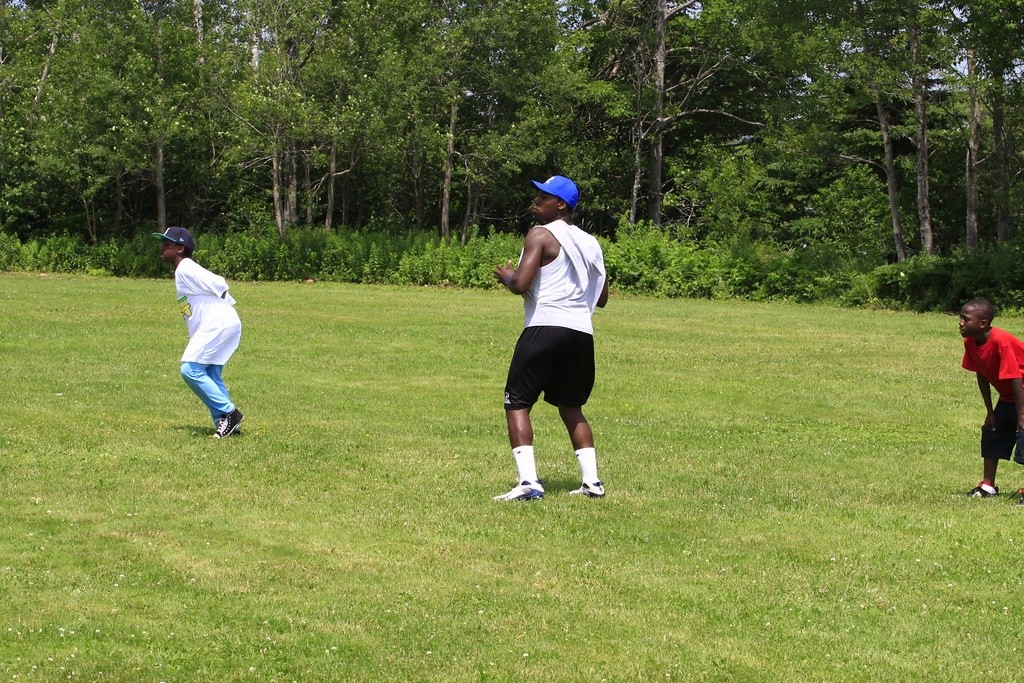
[952,292,1024,501]
[149,224,247,441]
[488,174,611,503]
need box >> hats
[531,175,580,209]
[151,226,195,255]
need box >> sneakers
[212,408,245,439]
[969,480,1000,499]
[1015,486,1024,507]
[568,481,606,499]
[492,477,545,503]
